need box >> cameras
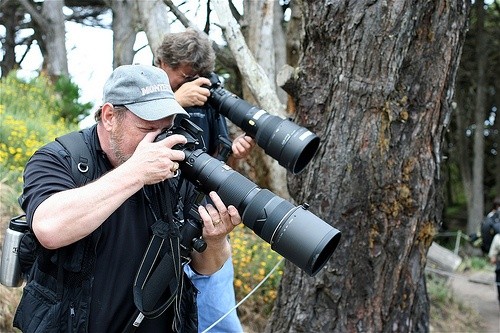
[177,71,320,175]
[153,117,342,276]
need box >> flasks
[0,214,28,287]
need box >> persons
[151,29,255,333]
[481,199,500,306]
[13,63,241,333]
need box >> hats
[100,64,190,122]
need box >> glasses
[176,68,199,81]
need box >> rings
[213,219,221,225]
